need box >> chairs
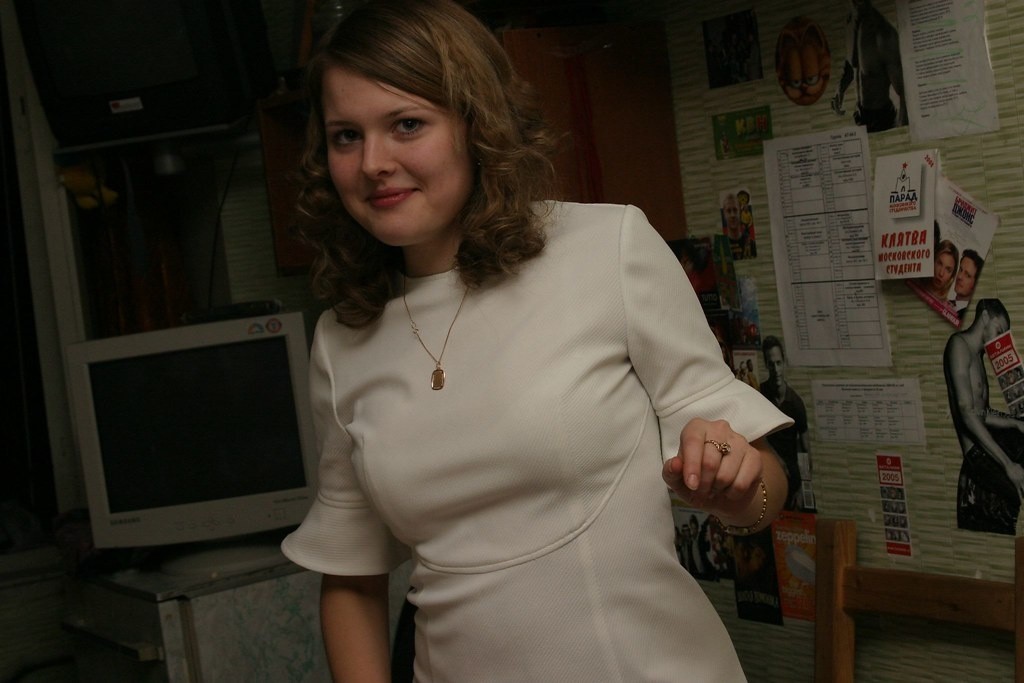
[815,519,1024,683]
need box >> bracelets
[713,479,768,535]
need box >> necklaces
[403,272,469,391]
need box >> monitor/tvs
[66,312,317,548]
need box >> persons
[917,220,984,320]
[759,336,813,512]
[831,0,909,132]
[674,512,710,580]
[281,0,795,683]
[722,189,756,260]
[943,299,1024,503]
[737,358,758,388]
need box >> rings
[704,440,731,455]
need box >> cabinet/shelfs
[257,19,687,272]
[59,544,385,683]
